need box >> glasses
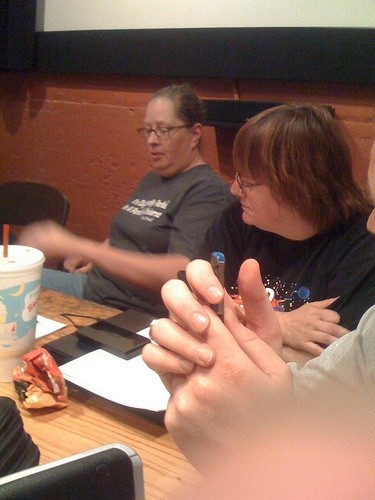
[237,172,263,194]
[137,125,193,137]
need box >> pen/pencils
[212,250,225,325]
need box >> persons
[19,83,231,319]
[140,143,375,479]
[190,102,375,356]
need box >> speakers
[6,0,38,71]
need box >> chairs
[0,181,69,269]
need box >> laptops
[42,309,168,425]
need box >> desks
[0,286,318,500]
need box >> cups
[0,244,46,383]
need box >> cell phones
[76,319,152,359]
